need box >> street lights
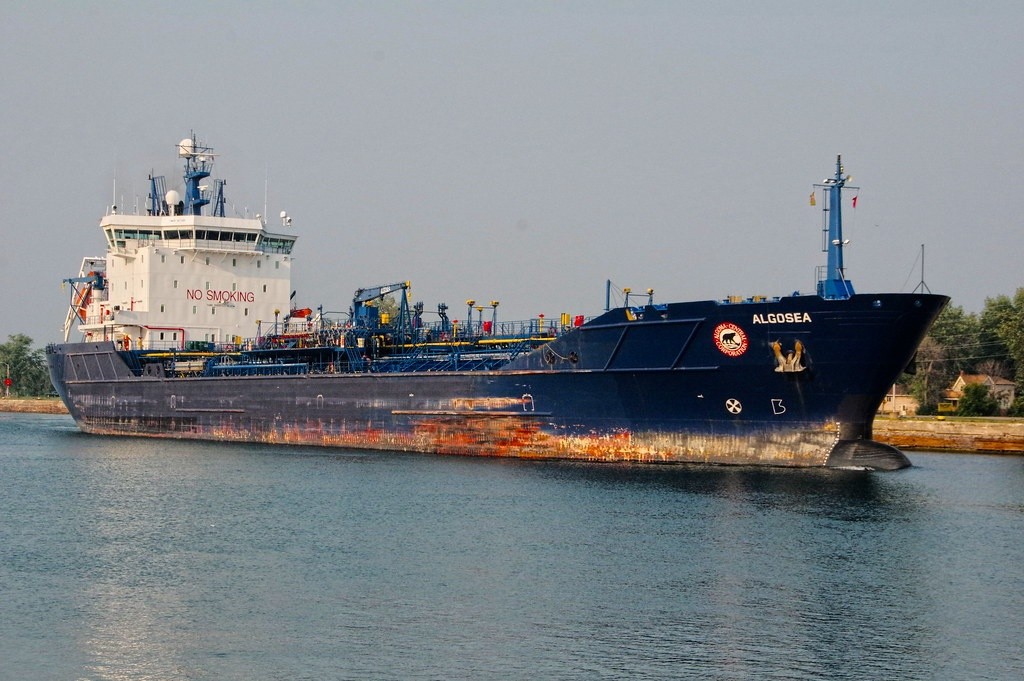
[0,362,10,397]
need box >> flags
[809,192,816,206]
[852,196,857,208]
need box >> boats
[46,128,952,471]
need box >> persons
[124,334,132,350]
